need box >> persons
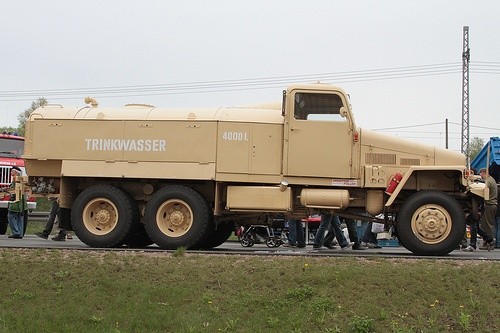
[281,218,306,249]
[313,214,355,250]
[34,201,66,241]
[0,165,28,239]
[465,169,496,251]
[478,168,498,250]
[323,216,368,250]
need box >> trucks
[21,86,490,255]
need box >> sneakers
[462,246,476,251]
[489,237,496,250]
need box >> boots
[479,240,489,250]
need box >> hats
[477,168,486,174]
[13,165,21,171]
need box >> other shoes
[284,240,384,250]
[8,234,22,239]
[52,232,65,241]
[35,231,49,239]
